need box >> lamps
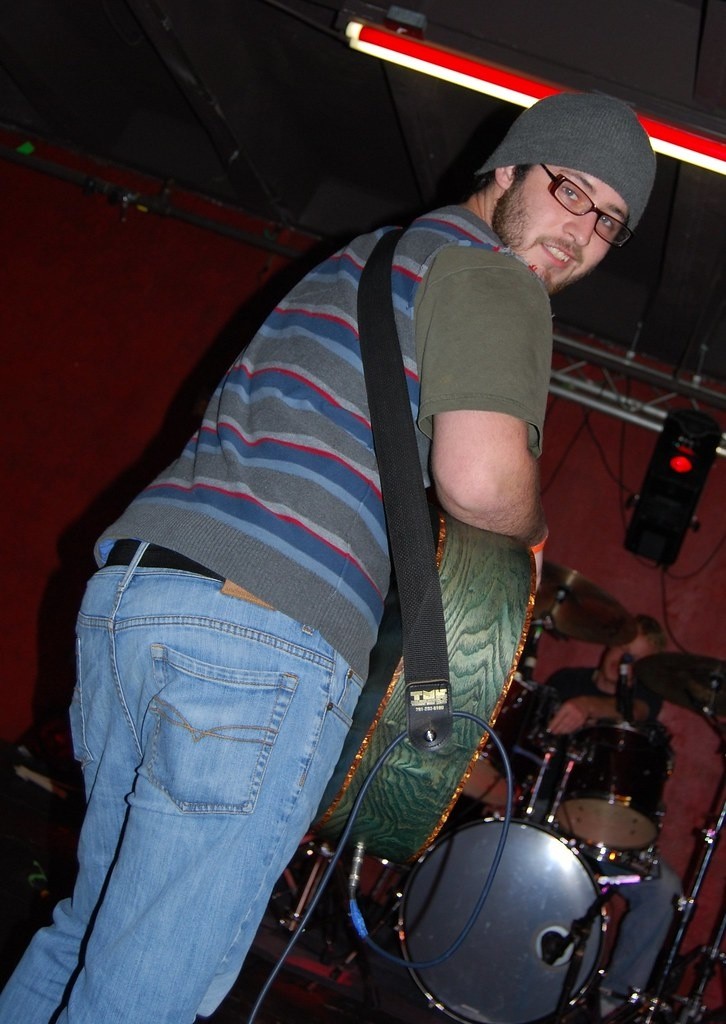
[348,15,725,176]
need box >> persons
[0,94,656,1024]
[499,613,682,1016]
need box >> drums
[309,509,539,864]
[399,817,608,1024]
[547,717,666,849]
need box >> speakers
[622,407,722,569]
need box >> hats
[474,92,657,236]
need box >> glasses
[539,163,637,250]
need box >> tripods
[599,804,726,1024]
[270,835,371,986]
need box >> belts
[104,539,228,582]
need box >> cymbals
[633,652,726,714]
[531,559,638,644]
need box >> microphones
[615,654,630,713]
[525,627,545,669]
[534,925,574,967]
